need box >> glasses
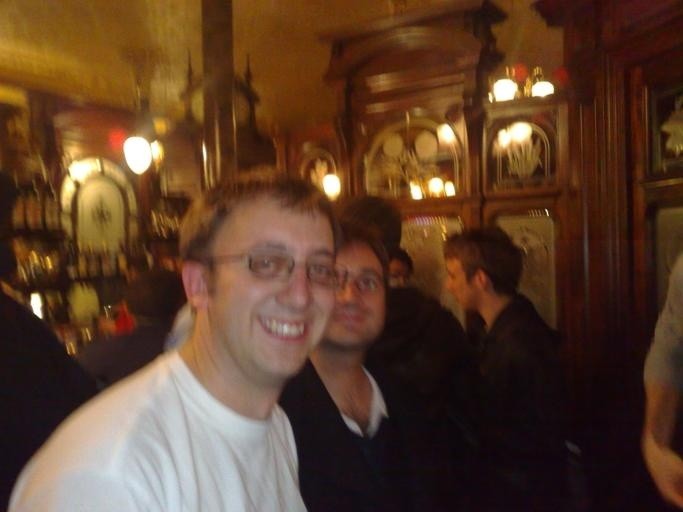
[350,269,380,293]
[198,252,347,295]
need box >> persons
[284,233,451,512]
[439,226,578,512]
[387,247,414,289]
[1,171,338,511]
[340,195,464,401]
[0,284,94,511]
[642,253,683,512]
